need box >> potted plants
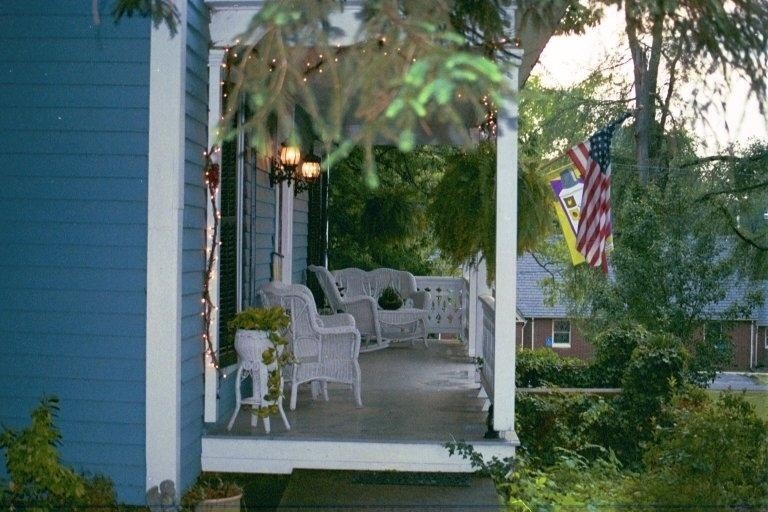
[225,305,302,435]
[179,470,245,512]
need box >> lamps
[267,128,321,199]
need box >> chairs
[258,263,438,412]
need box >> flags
[563,111,624,275]
[546,161,616,266]
